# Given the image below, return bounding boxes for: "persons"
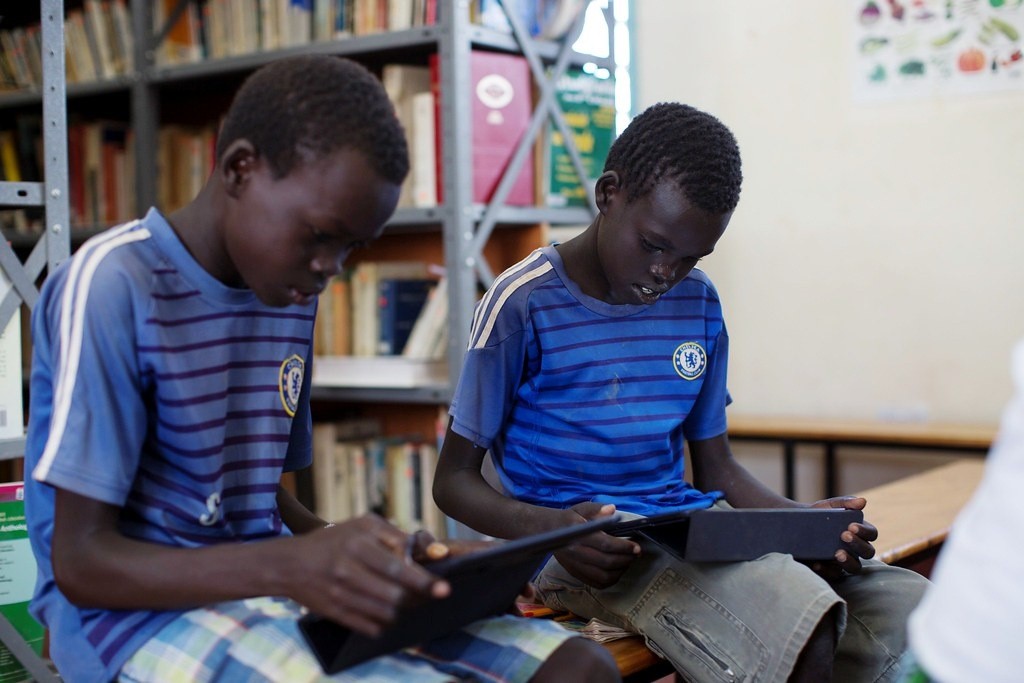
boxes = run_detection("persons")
[23,53,621,683]
[434,103,936,683]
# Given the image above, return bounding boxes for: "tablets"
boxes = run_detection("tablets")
[327,513,622,675]
[618,507,850,531]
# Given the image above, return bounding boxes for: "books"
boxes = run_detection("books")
[0,0,614,683]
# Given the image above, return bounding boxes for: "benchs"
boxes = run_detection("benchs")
[599,456,988,683]
[726,415,999,500]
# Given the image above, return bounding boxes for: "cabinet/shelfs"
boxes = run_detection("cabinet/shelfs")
[0,0,71,683]
[0,0,618,542]
